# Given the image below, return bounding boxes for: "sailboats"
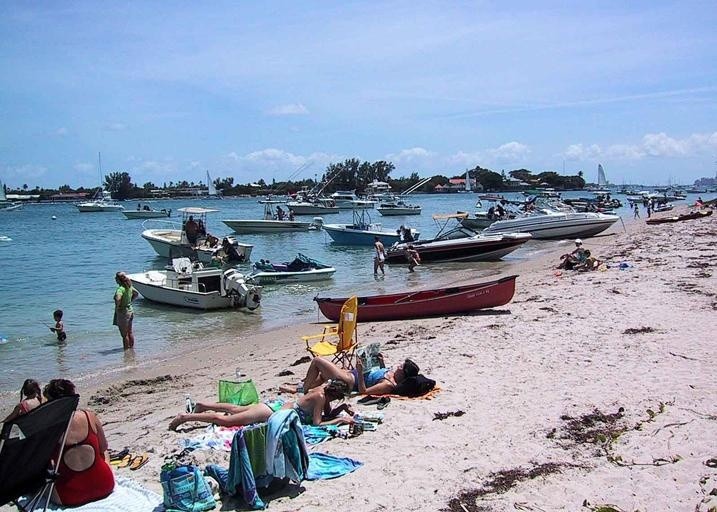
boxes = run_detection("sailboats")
[77,151,126,211]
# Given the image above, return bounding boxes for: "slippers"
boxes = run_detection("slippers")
[357,394,390,410]
[118,452,150,471]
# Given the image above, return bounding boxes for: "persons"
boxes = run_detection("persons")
[50,310,66,341]
[276,206,283,220]
[556,239,586,271]
[396,225,421,272]
[629,195,671,219]
[169,381,355,431]
[279,356,420,396]
[572,249,593,272]
[42,379,115,507]
[288,208,295,221]
[3,379,45,426]
[112,272,139,349]
[488,204,505,220]
[697,197,711,210]
[374,236,386,273]
[185,216,219,257]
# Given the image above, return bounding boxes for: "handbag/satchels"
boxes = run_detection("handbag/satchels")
[159,462,217,512]
[391,374,436,398]
[219,379,259,407]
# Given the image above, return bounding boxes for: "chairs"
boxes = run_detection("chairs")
[0,393,81,512]
[301,295,360,371]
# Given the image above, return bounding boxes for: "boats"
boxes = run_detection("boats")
[4,193,42,203]
[331,188,374,208]
[443,183,717,229]
[379,214,533,265]
[285,167,341,214]
[123,257,261,311]
[176,206,215,215]
[483,199,618,240]
[368,178,406,203]
[245,252,336,284]
[141,211,253,266]
[225,201,323,234]
[121,203,171,216]
[322,202,420,245]
[314,275,521,322]
